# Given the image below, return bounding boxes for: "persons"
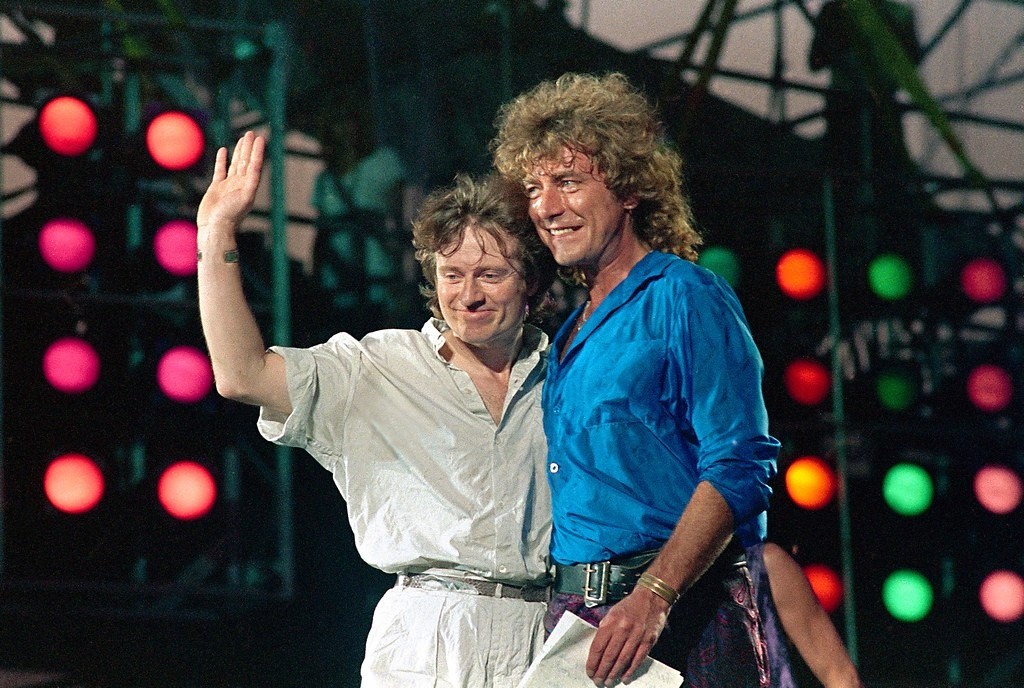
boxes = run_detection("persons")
[196,129,556,688]
[746,541,858,688]
[488,72,782,688]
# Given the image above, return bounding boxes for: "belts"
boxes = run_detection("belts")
[395,572,550,602]
[553,530,746,608]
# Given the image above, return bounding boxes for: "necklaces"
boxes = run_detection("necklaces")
[576,301,593,328]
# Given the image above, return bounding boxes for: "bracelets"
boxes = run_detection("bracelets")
[197,247,241,264]
[637,572,680,608]
